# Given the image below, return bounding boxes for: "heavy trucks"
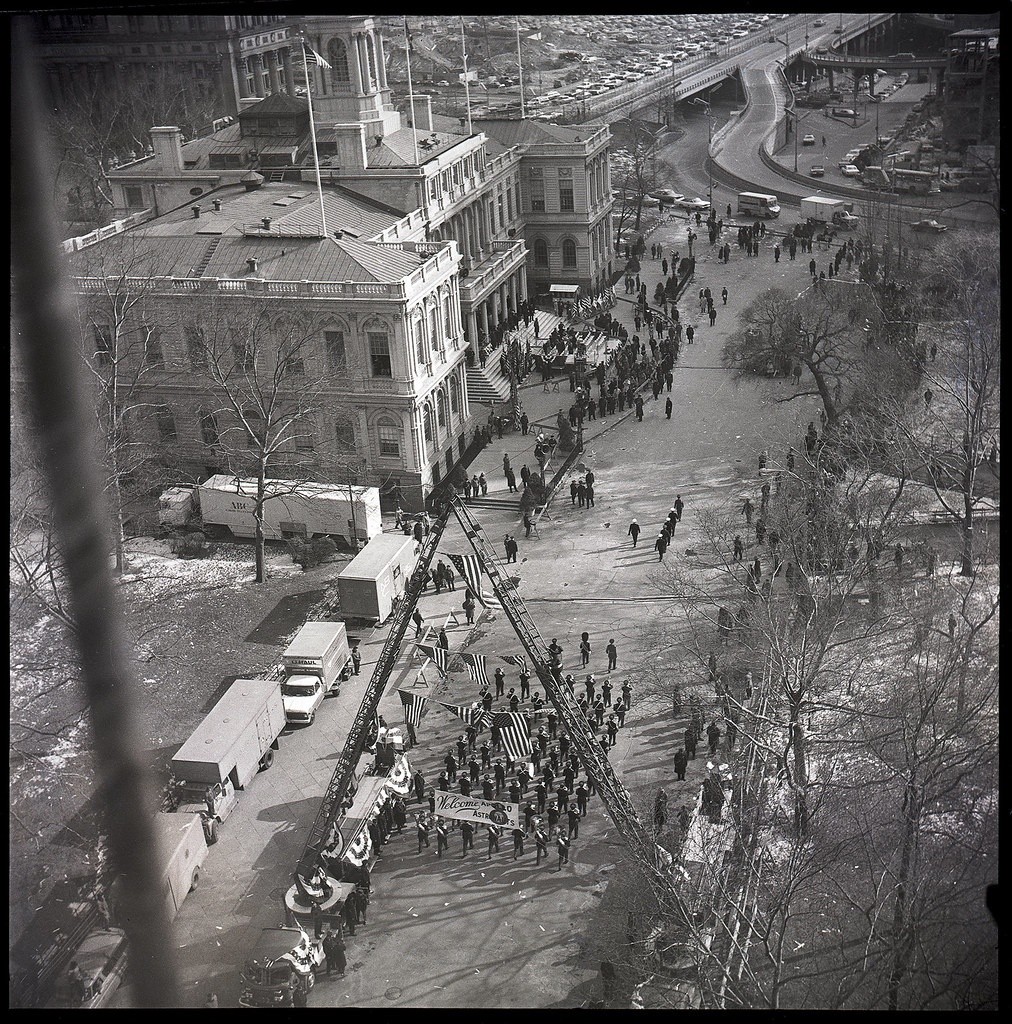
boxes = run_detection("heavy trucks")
[158,474,382,551]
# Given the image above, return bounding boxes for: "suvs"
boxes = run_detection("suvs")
[889,53,916,61]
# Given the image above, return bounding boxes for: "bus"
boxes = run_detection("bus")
[883,169,941,196]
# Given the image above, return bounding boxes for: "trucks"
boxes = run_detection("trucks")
[737,192,780,219]
[109,532,423,937]
[800,196,859,231]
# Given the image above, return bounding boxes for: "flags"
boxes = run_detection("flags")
[396,687,429,730]
[496,653,531,677]
[487,712,534,761]
[302,40,334,69]
[442,551,487,609]
[456,649,490,689]
[416,642,449,681]
[437,701,495,729]
[404,20,412,49]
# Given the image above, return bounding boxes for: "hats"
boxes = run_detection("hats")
[417,632,630,838]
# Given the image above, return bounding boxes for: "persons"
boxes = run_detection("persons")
[344,856,371,935]
[821,135,827,146]
[946,612,959,644]
[366,794,406,855]
[352,646,363,676]
[311,901,324,939]
[321,929,347,976]
[627,404,937,638]
[770,216,938,402]
[464,192,763,427]
[378,713,388,727]
[200,990,219,1007]
[406,555,725,875]
[205,785,218,819]
[389,410,595,636]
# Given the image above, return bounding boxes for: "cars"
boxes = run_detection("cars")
[835,109,858,117]
[523,51,673,118]
[648,190,683,203]
[909,220,947,234]
[625,195,659,207]
[839,143,881,182]
[674,197,710,211]
[879,92,933,147]
[802,134,814,145]
[810,165,824,176]
[828,69,908,99]
[468,14,845,59]
[388,75,513,97]
[42,927,128,1009]
